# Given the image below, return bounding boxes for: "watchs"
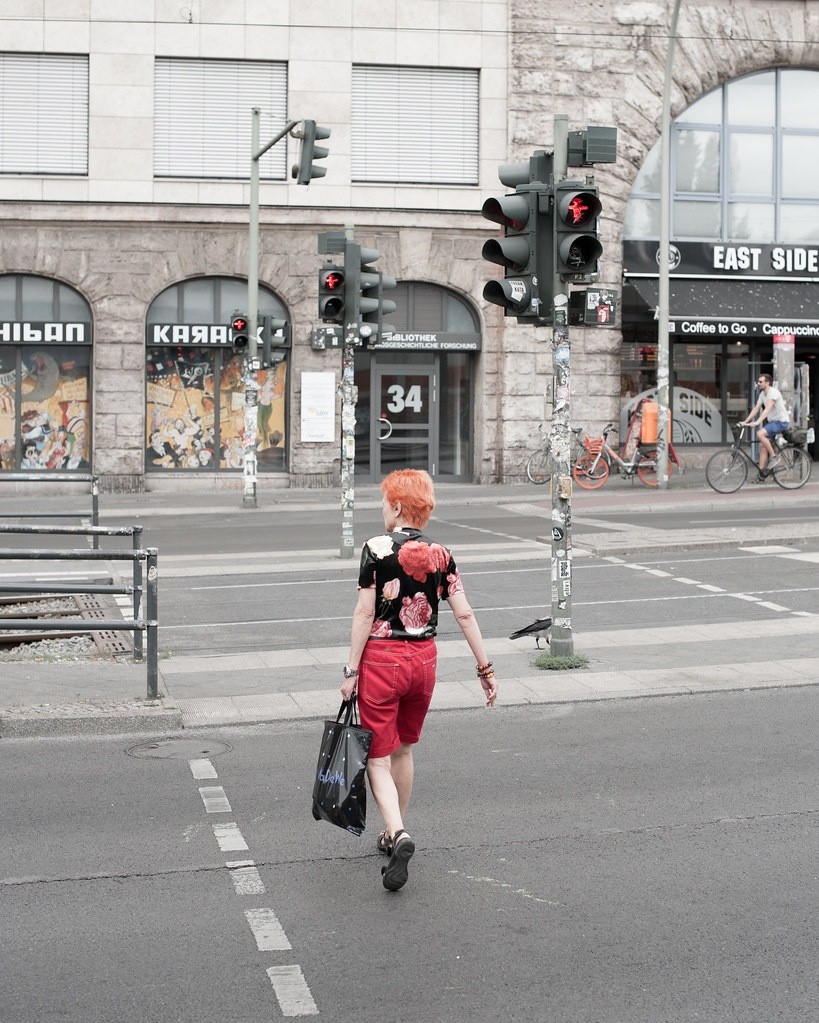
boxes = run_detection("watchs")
[343,666,359,678]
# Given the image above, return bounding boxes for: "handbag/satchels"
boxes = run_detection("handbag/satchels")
[311,692,373,836]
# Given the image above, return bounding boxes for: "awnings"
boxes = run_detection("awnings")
[628,277,819,337]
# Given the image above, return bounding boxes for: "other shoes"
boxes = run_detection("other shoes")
[767,455,781,470]
[752,477,764,484]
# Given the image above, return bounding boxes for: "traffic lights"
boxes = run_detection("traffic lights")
[363,269,395,345]
[290,119,328,186]
[551,175,603,276]
[260,315,288,369]
[481,150,540,319]
[230,310,247,354]
[345,242,379,329]
[318,264,344,320]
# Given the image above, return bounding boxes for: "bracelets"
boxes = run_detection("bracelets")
[475,662,494,677]
[755,421,760,426]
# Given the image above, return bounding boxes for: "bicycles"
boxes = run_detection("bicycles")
[573,424,679,490]
[705,421,813,495]
[526,425,611,483]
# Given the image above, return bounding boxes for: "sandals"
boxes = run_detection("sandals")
[381,830,415,891]
[376,831,396,858]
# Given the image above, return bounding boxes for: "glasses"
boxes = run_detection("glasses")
[757,380,764,384]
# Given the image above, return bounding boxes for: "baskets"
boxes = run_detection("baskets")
[585,434,606,454]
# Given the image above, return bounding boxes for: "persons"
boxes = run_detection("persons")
[741,374,790,484]
[340,469,499,891]
